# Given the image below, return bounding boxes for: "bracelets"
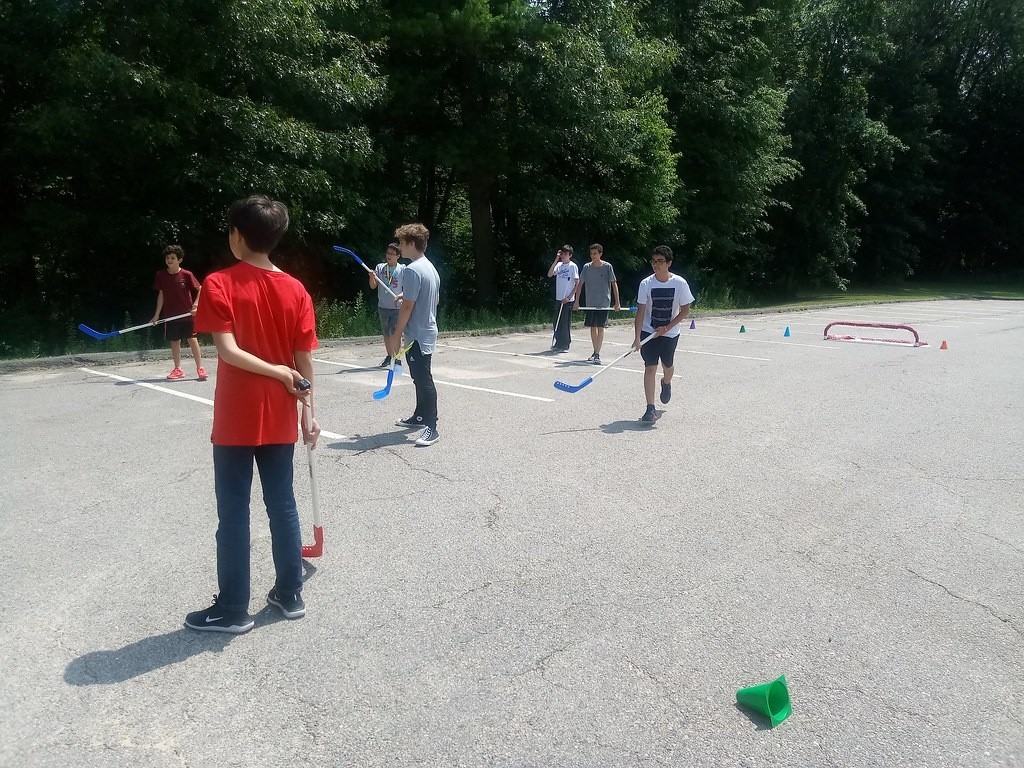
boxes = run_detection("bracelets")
[665,326,668,332]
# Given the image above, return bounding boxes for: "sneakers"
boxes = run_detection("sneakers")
[593,354,601,365]
[660,377,671,404]
[642,404,658,421]
[268,586,306,618]
[558,345,569,352]
[184,594,255,633]
[167,368,185,379]
[415,428,440,446]
[196,367,208,379]
[396,413,425,428]
[588,353,601,361]
[551,345,560,351]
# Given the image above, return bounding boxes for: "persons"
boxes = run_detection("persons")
[631,246,695,422]
[388,224,441,446]
[369,244,406,366]
[150,245,208,378]
[573,244,620,364]
[185,195,321,632]
[548,246,580,352]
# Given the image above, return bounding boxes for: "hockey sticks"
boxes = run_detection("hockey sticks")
[332,245,401,304]
[551,276,570,352]
[577,305,638,313]
[554,330,657,394]
[298,378,325,558]
[78,312,191,341]
[372,355,395,400]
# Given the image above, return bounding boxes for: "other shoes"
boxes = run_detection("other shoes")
[382,356,391,365]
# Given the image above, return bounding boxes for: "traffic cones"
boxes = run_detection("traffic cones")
[940,340,948,349]
[689,320,695,329]
[736,675,792,729]
[784,327,791,336]
[739,325,746,332]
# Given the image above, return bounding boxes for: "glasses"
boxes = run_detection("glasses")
[386,252,398,256]
[650,259,666,265]
